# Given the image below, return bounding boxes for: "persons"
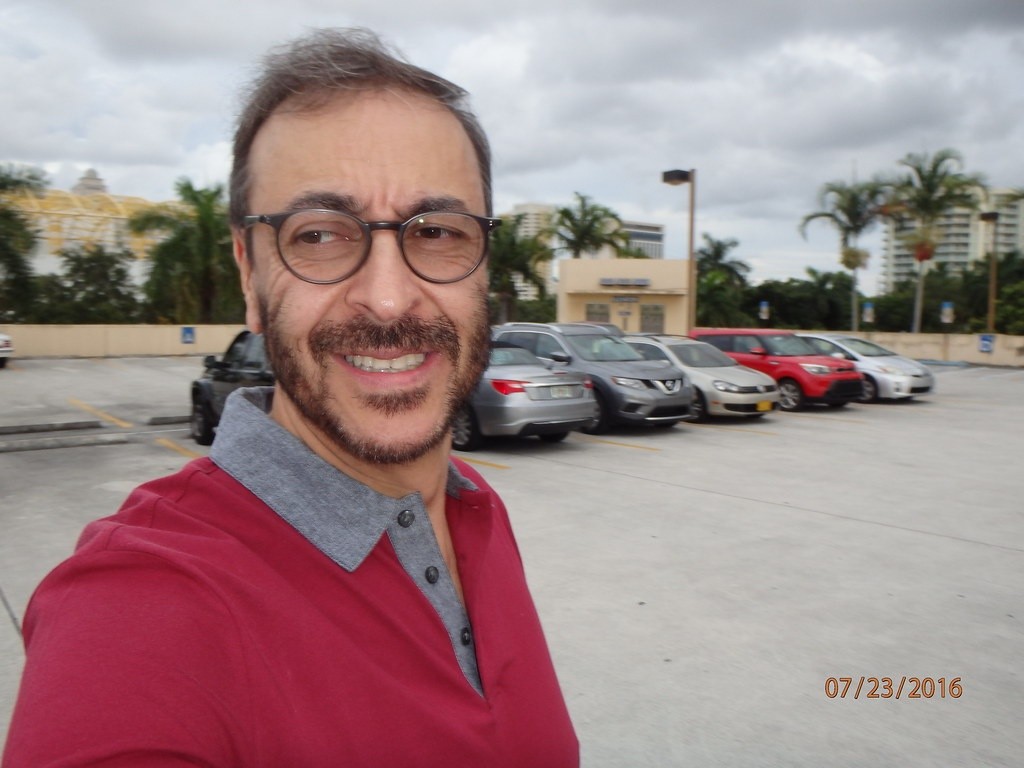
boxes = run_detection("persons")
[1,32,580,767]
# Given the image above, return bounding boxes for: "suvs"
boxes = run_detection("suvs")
[489,320,698,434]
[684,326,865,410]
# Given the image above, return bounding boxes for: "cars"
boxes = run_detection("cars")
[186,327,276,445]
[450,340,596,452]
[795,330,934,406]
[594,332,783,423]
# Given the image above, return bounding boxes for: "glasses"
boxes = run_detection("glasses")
[242,207,503,284]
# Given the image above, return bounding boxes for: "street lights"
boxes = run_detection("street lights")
[661,169,697,339]
